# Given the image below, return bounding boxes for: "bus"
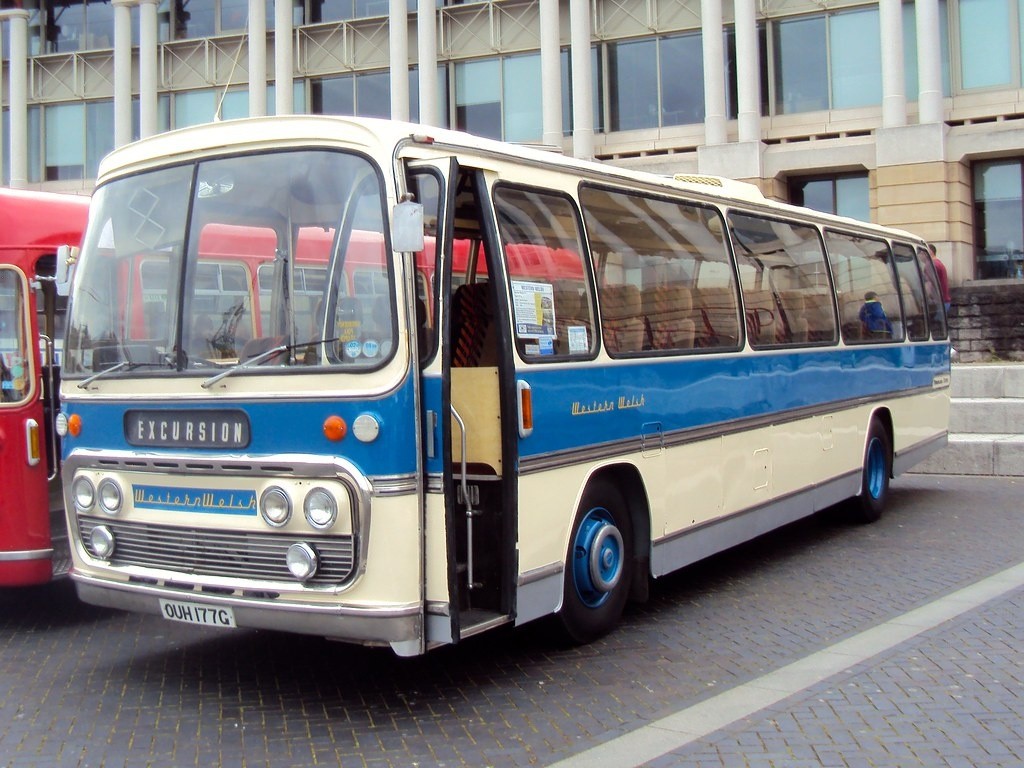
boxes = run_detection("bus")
[0,187,612,616]
[50,111,956,665]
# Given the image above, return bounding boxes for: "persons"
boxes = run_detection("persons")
[858,291,895,339]
[919,244,951,321]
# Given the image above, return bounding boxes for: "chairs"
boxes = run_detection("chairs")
[449,283,852,365]
[238,297,432,366]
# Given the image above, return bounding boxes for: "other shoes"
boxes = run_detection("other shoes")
[950,347,957,358]
